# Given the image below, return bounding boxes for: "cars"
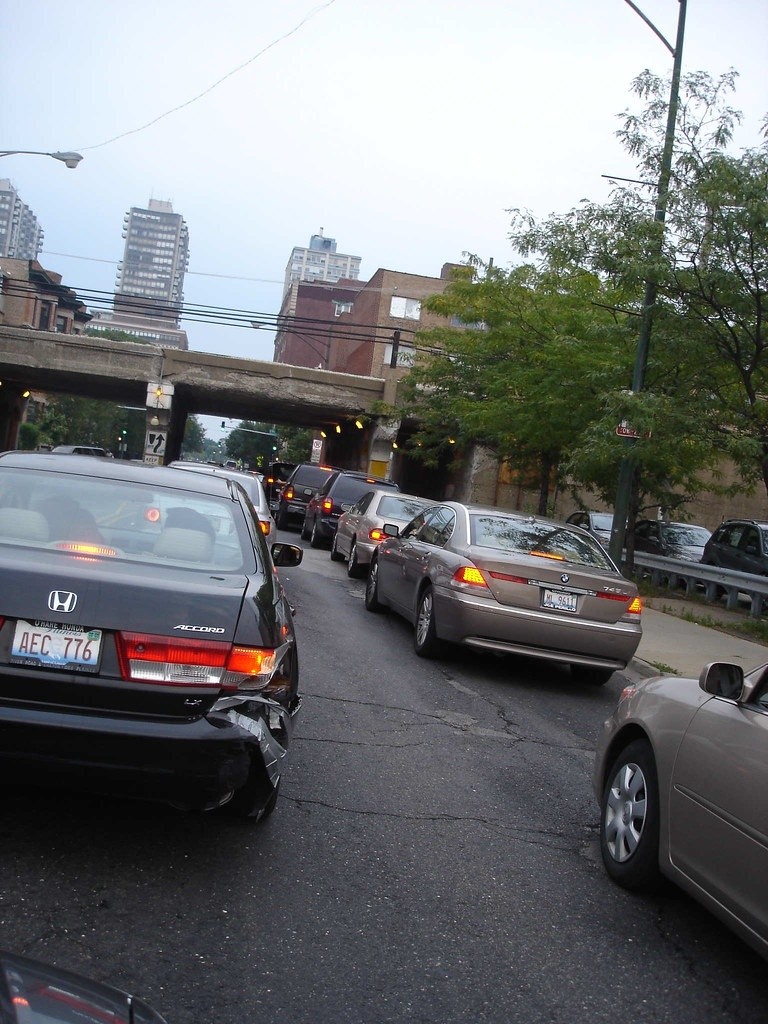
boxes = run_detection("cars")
[140,460,277,574]
[366,501,643,684]
[331,489,443,578]
[593,663,768,963]
[623,518,713,587]
[1,448,304,823]
[566,510,614,549]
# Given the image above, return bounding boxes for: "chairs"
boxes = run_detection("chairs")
[746,535,767,556]
[152,528,215,563]
[0,507,51,543]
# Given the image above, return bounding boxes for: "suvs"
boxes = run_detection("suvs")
[51,445,108,458]
[701,518,768,611]
[300,469,400,548]
[207,460,343,529]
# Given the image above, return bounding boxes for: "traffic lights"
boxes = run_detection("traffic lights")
[221,421,225,432]
[219,450,222,454]
[217,441,222,448]
[271,440,278,454]
[122,424,128,436]
[213,450,216,455]
[117,435,122,442]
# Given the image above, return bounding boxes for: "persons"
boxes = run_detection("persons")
[163,506,216,545]
